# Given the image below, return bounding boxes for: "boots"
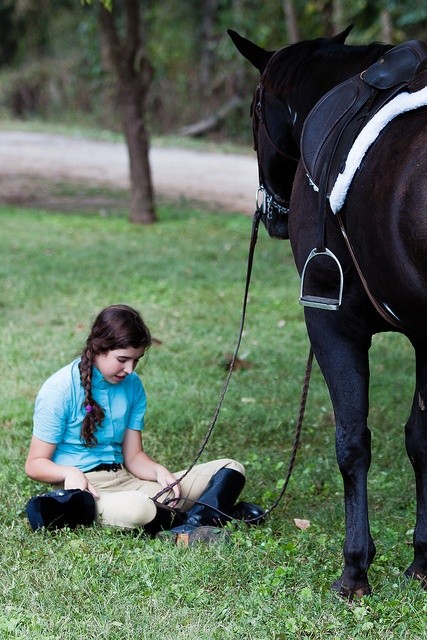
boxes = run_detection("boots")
[154,467,246,550]
[133,497,266,534]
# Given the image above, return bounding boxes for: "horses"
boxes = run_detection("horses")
[226,21,427,601]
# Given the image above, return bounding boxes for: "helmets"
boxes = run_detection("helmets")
[25,489,95,532]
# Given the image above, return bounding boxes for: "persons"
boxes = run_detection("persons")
[24,303,264,543]
